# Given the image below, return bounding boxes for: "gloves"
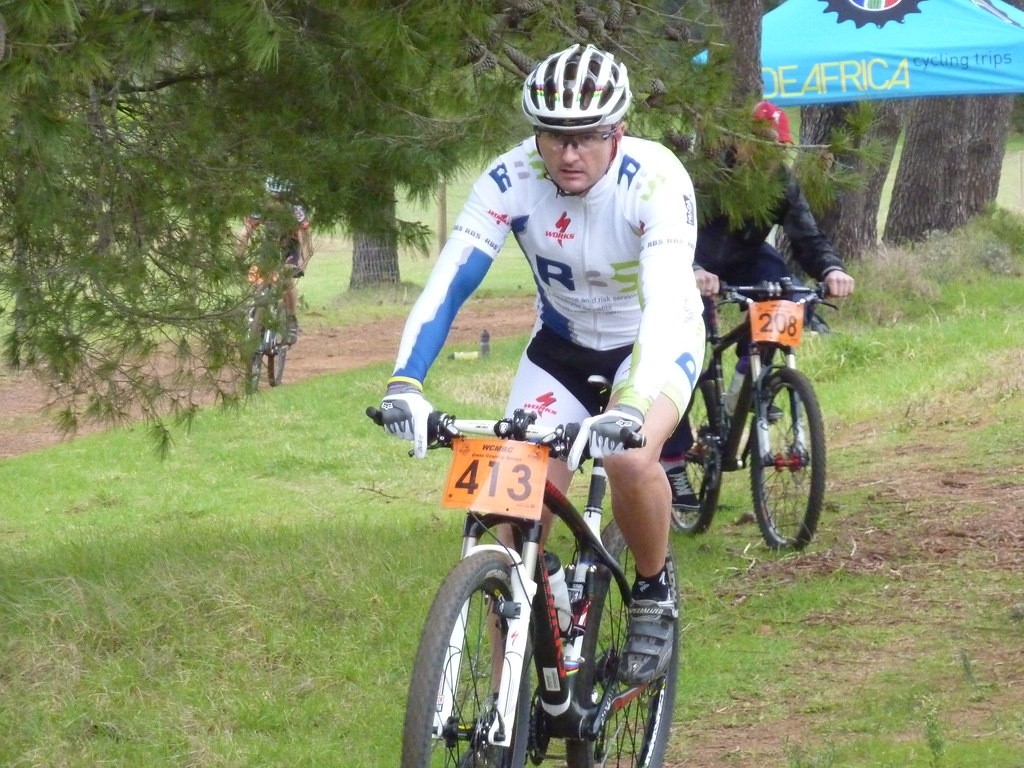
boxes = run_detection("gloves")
[567,404,644,471]
[380,382,433,459]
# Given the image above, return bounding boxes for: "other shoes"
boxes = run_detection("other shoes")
[286,324,298,344]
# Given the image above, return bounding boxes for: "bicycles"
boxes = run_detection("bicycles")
[244,258,304,396]
[365,374,680,768]
[656,277,841,553]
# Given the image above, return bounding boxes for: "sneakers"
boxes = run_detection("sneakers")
[666,466,700,509]
[458,748,474,768]
[621,589,678,685]
[750,400,784,419]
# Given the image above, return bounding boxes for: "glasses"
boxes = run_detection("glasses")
[534,126,617,154]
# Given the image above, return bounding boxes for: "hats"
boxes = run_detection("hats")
[751,102,793,144]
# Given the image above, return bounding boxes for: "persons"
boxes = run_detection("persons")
[237,172,309,348]
[660,100,855,512]
[382,42,708,686]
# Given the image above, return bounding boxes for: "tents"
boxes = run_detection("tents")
[646,0,1024,108]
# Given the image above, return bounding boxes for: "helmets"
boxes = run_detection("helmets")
[522,44,632,130]
[265,173,295,194]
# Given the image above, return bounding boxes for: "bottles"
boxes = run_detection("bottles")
[543,549,573,635]
[447,351,479,360]
[725,353,749,416]
[560,595,588,665]
[480,329,490,358]
